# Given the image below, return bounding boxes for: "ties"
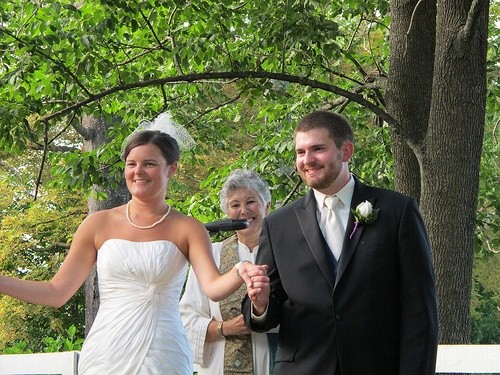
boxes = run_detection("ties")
[324,196,343,261]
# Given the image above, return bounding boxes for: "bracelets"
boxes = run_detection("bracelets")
[217,322,225,339]
[236,260,252,277]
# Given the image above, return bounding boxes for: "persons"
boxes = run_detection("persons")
[0,111,264,375]
[178,169,271,375]
[248,110,438,375]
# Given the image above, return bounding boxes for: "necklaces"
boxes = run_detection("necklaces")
[124,200,171,229]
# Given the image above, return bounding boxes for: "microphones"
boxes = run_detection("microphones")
[204,218,249,233]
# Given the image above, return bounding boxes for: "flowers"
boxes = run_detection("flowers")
[350,200,381,239]
